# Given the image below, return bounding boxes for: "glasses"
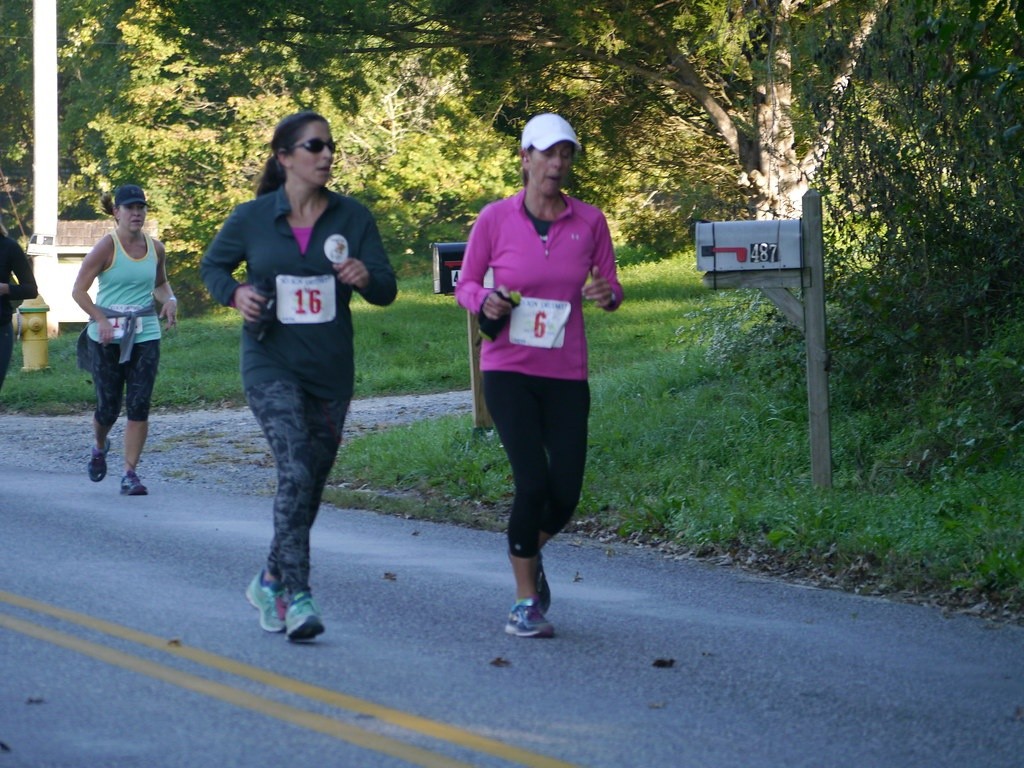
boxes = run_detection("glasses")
[287,139,335,153]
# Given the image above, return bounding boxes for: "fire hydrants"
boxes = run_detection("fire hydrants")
[10,292,53,373]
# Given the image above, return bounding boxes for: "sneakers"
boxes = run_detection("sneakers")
[245,568,289,633]
[508,546,551,614]
[120,470,147,495]
[505,593,554,638]
[285,591,325,641]
[87,437,110,482]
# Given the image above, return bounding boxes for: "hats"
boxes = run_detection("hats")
[522,114,581,152]
[115,185,150,207]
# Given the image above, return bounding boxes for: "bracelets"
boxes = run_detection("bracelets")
[603,290,617,309]
[169,297,176,301]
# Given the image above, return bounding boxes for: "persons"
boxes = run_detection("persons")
[72,184,178,495]
[455,113,624,638]
[0,224,38,392]
[198,112,398,644]
